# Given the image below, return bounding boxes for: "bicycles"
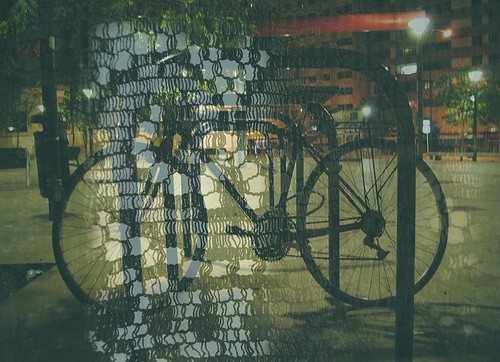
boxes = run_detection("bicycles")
[48,53,448,315]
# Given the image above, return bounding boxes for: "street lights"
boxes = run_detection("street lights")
[408,18,427,164]
[466,66,483,162]
[429,28,453,155]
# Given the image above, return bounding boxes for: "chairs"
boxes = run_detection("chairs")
[0,147,32,188]
